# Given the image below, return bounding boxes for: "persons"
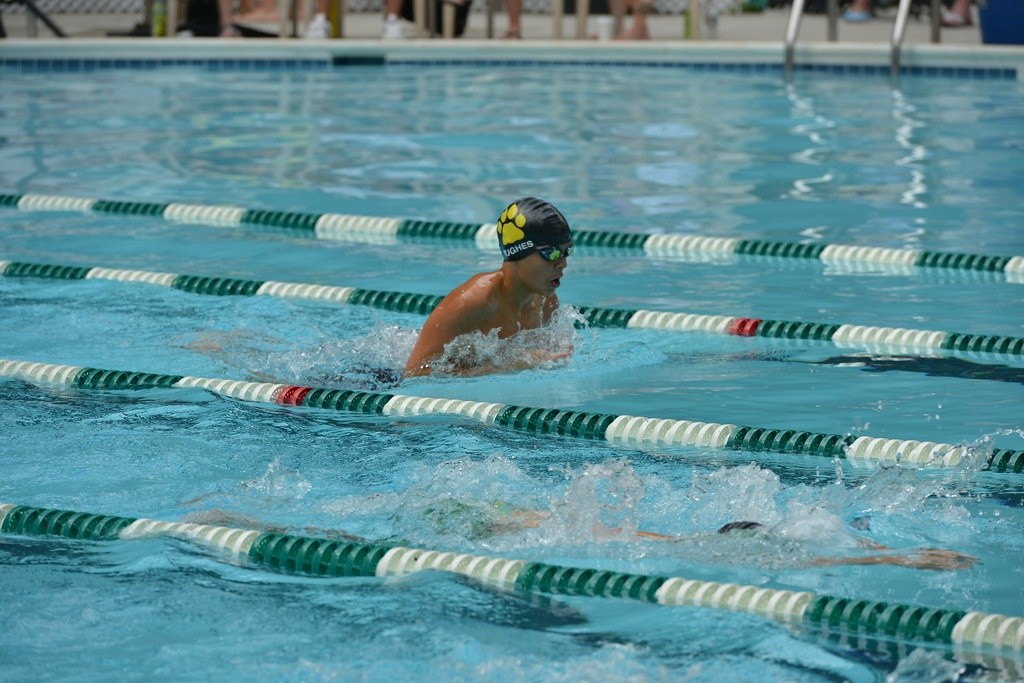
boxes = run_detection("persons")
[169,491,981,571]
[176,196,574,382]
[0,0,972,41]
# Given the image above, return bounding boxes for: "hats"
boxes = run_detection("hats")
[496,197,572,261]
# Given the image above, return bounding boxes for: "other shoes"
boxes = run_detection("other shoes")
[382,13,408,39]
[307,13,334,37]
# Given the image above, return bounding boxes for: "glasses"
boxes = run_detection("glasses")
[535,243,575,261]
